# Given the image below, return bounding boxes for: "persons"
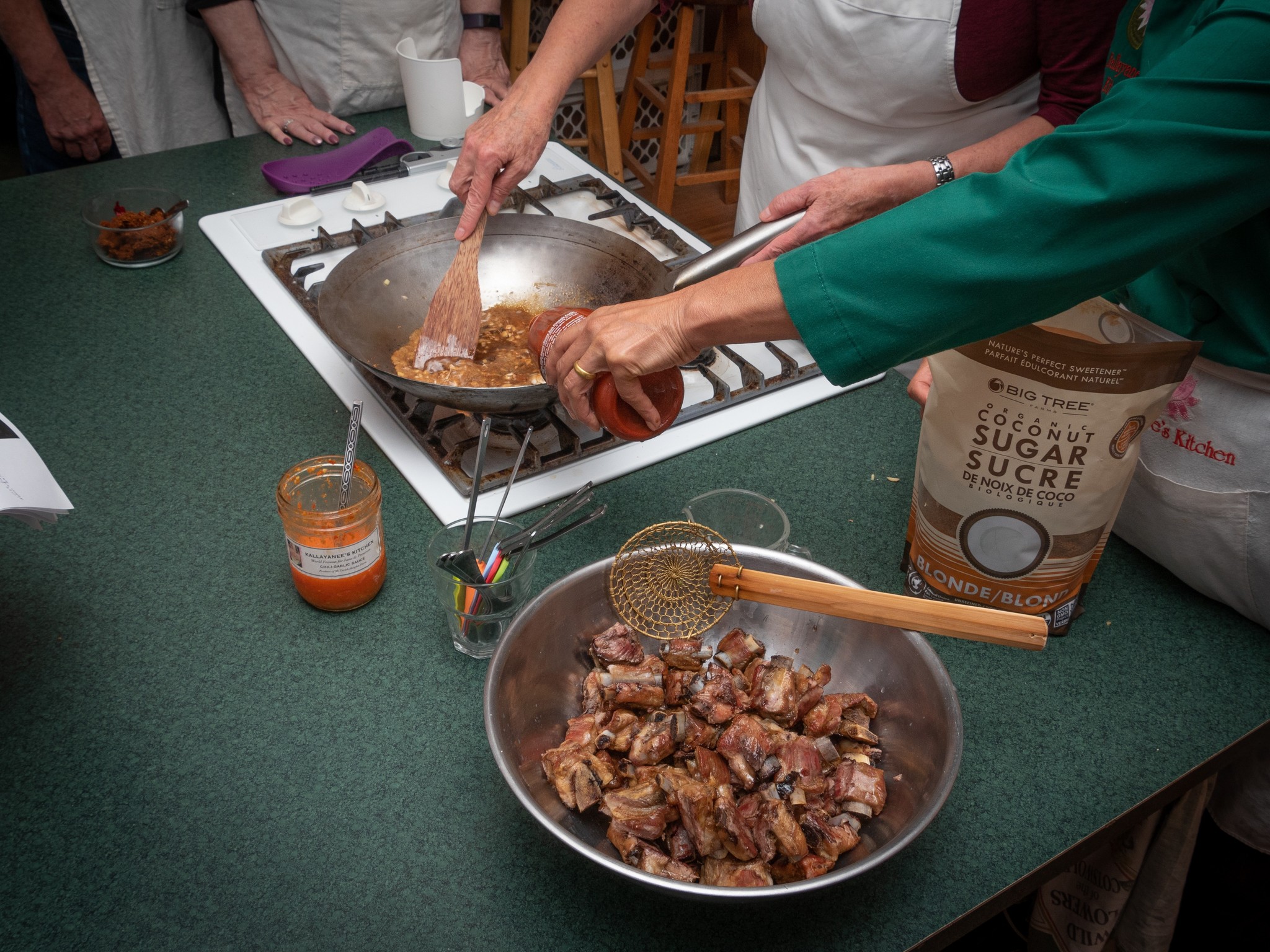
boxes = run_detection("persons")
[544,1,1270,952]
[0,0,514,178]
[450,0,1124,270]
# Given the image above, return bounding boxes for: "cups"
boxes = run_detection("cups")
[681,488,813,562]
[427,516,538,659]
[276,455,387,611]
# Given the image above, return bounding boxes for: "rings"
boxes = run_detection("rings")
[574,359,597,379]
[284,118,294,133]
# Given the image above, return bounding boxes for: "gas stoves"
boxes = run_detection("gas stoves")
[198,141,887,529]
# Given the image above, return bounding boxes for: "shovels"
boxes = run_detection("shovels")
[413,208,487,370]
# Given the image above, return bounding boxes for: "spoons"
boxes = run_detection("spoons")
[149,199,190,223]
[435,549,519,647]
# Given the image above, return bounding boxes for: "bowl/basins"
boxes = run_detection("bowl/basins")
[483,542,963,897]
[79,187,186,268]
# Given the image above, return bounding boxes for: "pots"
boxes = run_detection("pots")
[317,208,808,413]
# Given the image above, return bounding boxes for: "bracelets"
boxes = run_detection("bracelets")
[462,11,501,29]
[927,155,955,186]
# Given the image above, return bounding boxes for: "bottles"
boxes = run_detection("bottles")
[527,305,685,442]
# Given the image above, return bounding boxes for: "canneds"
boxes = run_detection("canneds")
[276,455,387,612]
